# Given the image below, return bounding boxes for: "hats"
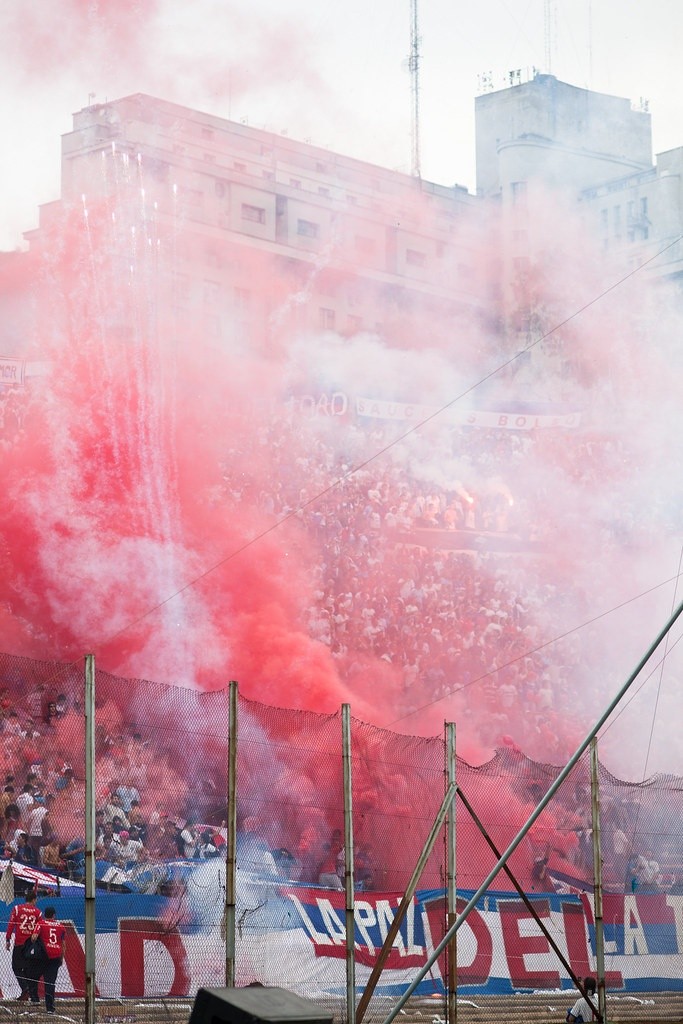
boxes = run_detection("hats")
[129,825,142,832]
[534,856,543,865]
[119,831,130,839]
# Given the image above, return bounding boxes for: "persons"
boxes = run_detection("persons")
[566,977,598,1024]
[1,429,682,891]
[5,891,68,1013]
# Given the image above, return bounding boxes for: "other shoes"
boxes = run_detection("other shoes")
[47,1007,54,1014]
[30,999,41,1005]
[17,992,28,1001]
